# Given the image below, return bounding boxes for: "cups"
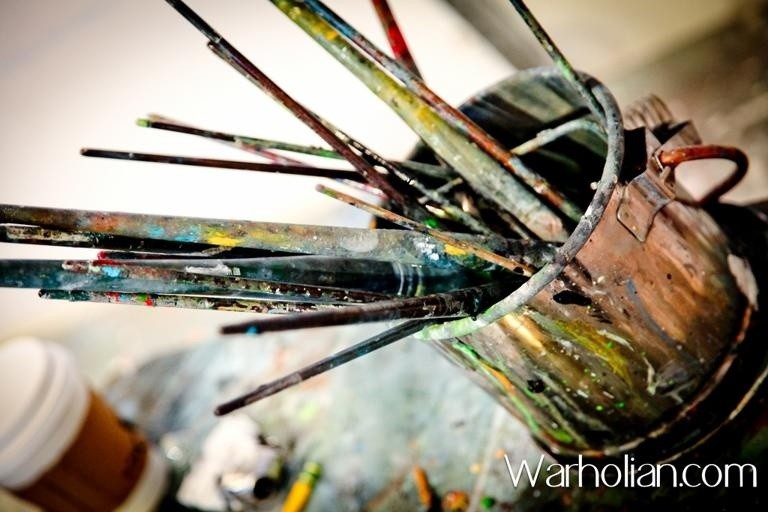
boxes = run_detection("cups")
[364,64,768,482]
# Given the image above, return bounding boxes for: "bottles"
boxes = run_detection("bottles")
[0,338,170,512]
